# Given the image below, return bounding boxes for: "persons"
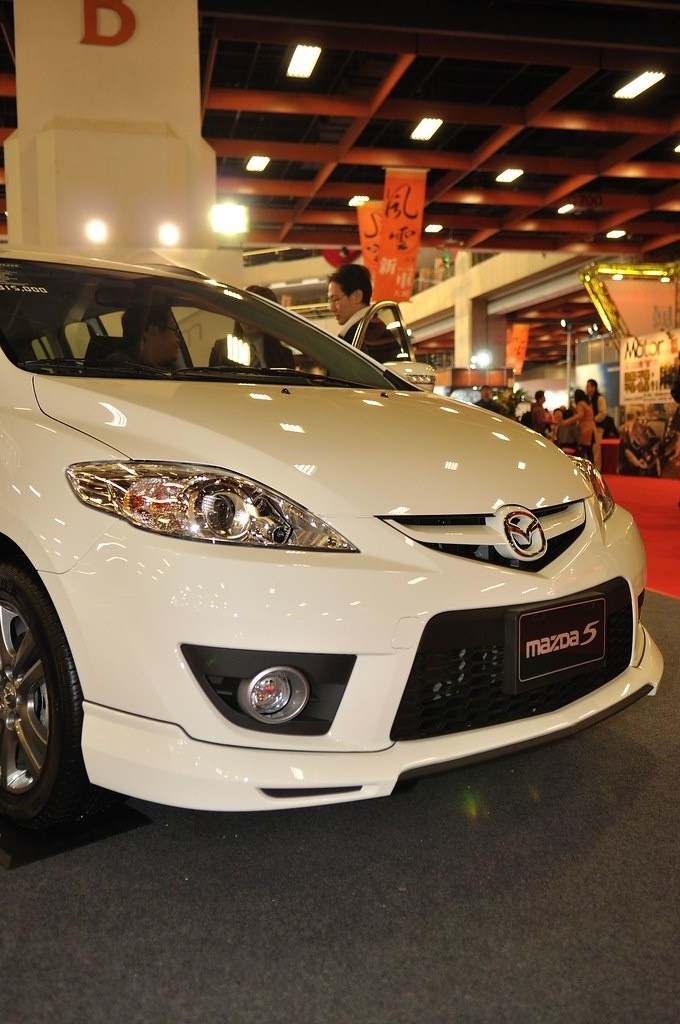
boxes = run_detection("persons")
[560,389,597,464]
[327,264,404,362]
[208,284,295,369]
[671,370,680,434]
[531,391,550,435]
[619,416,656,476]
[109,301,180,369]
[473,385,502,413]
[544,406,574,432]
[586,379,618,439]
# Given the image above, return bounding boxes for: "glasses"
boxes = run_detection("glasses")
[328,293,346,303]
[167,325,180,338]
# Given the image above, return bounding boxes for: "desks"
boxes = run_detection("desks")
[601,437,623,475]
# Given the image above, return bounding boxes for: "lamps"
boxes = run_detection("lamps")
[561,318,569,329]
[345,194,372,211]
[493,164,527,185]
[243,151,273,176]
[587,322,600,337]
[279,36,325,86]
[608,66,670,104]
[406,113,445,142]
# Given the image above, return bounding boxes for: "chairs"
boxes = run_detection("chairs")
[555,426,590,463]
[82,338,137,377]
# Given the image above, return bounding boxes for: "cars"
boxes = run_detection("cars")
[0,249,664,827]
